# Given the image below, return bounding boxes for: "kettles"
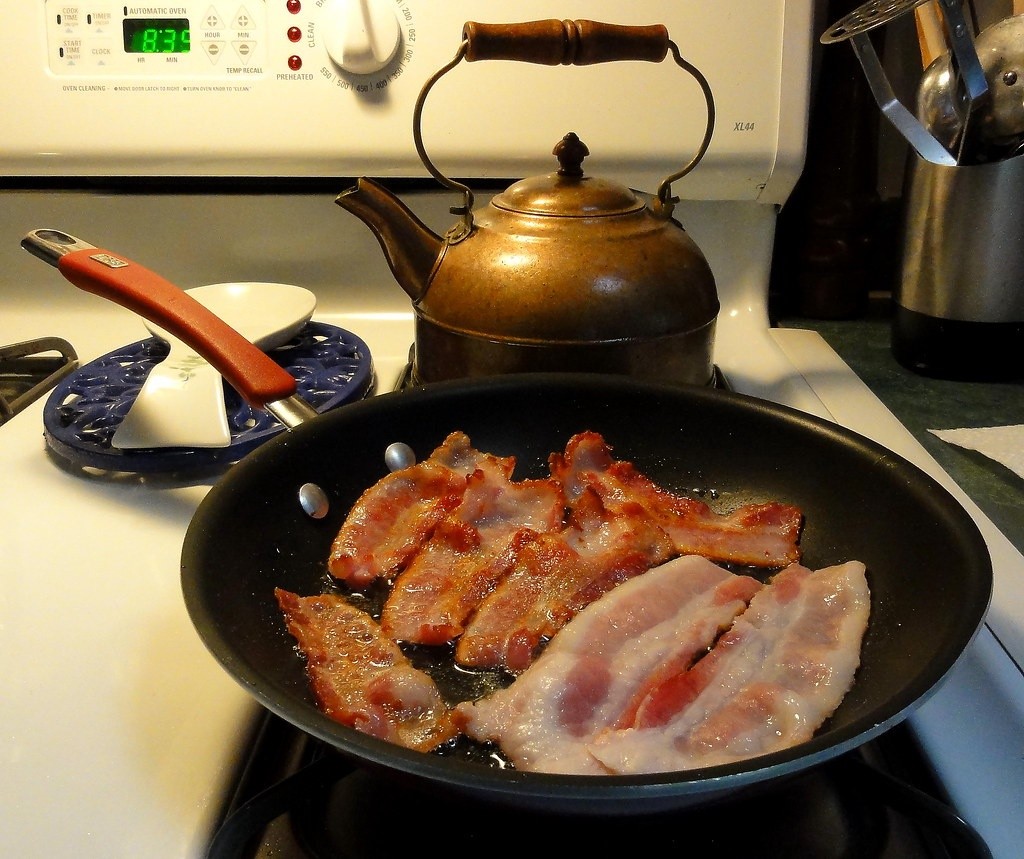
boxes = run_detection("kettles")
[333,21,721,387]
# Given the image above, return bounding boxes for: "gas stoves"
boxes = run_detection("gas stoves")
[0,0,1024,859]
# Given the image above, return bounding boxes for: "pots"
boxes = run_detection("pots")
[19,229,991,816]
